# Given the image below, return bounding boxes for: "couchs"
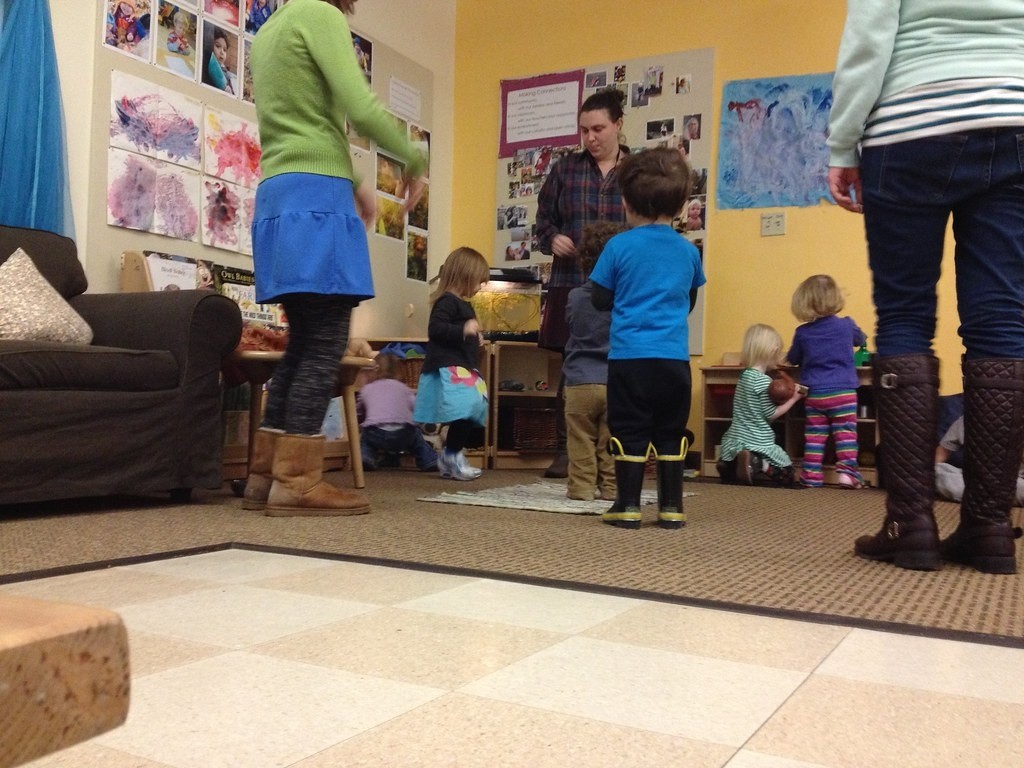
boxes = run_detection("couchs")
[0,224,243,507]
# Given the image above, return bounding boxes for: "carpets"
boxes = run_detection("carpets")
[0,457,1024,648]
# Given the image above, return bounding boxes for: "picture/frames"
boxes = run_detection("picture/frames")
[102,0,433,284]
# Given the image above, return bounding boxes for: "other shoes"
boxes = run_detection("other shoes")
[715,449,763,486]
[835,473,863,489]
[437,444,481,481]
[544,452,568,478]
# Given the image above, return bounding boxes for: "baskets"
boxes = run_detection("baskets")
[397,358,425,388]
[511,403,561,453]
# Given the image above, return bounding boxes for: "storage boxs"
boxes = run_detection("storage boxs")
[399,358,424,388]
[511,405,558,449]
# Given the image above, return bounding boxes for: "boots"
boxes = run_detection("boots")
[854,353,941,570]
[940,353,1024,574]
[601,436,686,529]
[243,426,371,517]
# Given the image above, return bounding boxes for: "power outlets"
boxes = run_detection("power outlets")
[761,211,787,236]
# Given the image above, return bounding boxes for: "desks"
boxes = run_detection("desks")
[238,350,376,488]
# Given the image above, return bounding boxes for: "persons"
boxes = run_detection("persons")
[826,0,1024,575]
[785,274,869,490]
[715,323,808,488]
[241,1,430,518]
[498,64,705,263]
[352,38,433,280]
[535,86,707,527]
[355,352,439,473]
[106,0,278,100]
[934,414,1024,509]
[412,247,492,482]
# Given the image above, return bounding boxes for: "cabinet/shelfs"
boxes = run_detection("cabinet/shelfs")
[699,364,881,489]
[492,334,571,474]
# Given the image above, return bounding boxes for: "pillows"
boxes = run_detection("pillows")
[0,246,95,345]
